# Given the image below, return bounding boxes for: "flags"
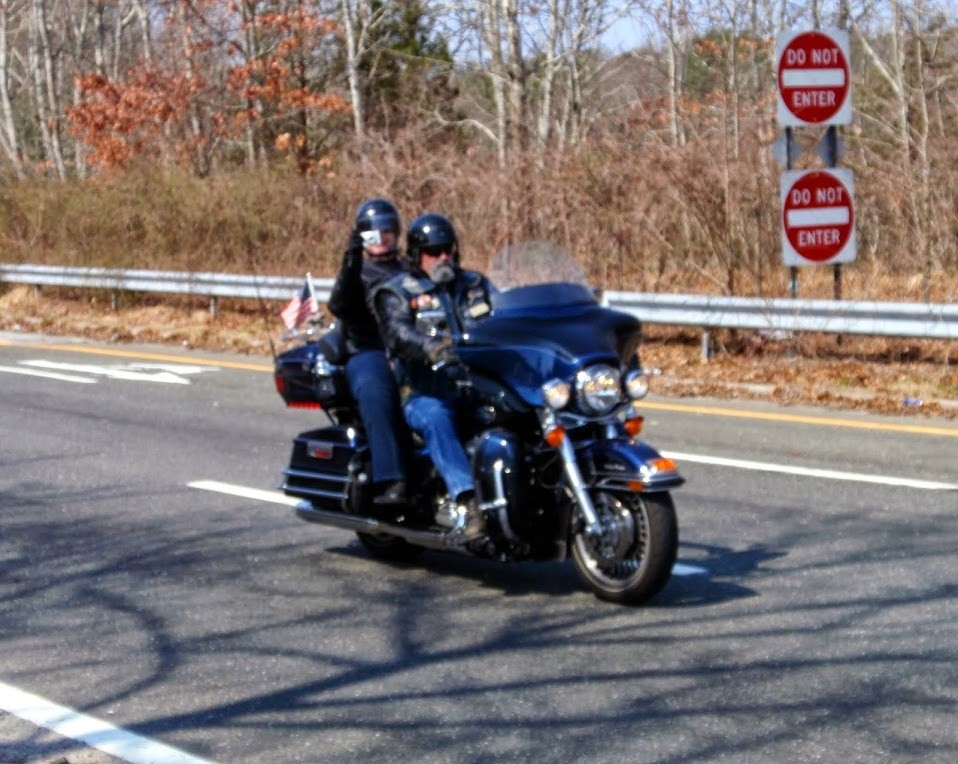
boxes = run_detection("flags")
[279,278,323,335]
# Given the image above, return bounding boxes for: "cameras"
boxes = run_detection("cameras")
[359,229,382,247]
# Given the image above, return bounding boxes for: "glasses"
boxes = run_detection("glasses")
[423,244,456,257]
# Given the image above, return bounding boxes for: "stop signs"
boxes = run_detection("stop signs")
[773,27,853,128]
[778,168,858,264]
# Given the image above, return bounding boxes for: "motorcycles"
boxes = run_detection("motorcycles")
[274,243,686,607]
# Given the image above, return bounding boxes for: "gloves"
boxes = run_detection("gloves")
[422,338,458,367]
[347,230,364,271]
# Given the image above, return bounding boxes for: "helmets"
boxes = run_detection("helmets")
[355,200,400,231]
[406,213,460,258]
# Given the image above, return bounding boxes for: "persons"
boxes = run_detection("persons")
[327,198,426,500]
[369,214,505,544]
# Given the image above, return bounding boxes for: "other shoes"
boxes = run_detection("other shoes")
[454,496,487,545]
[383,480,408,500]
